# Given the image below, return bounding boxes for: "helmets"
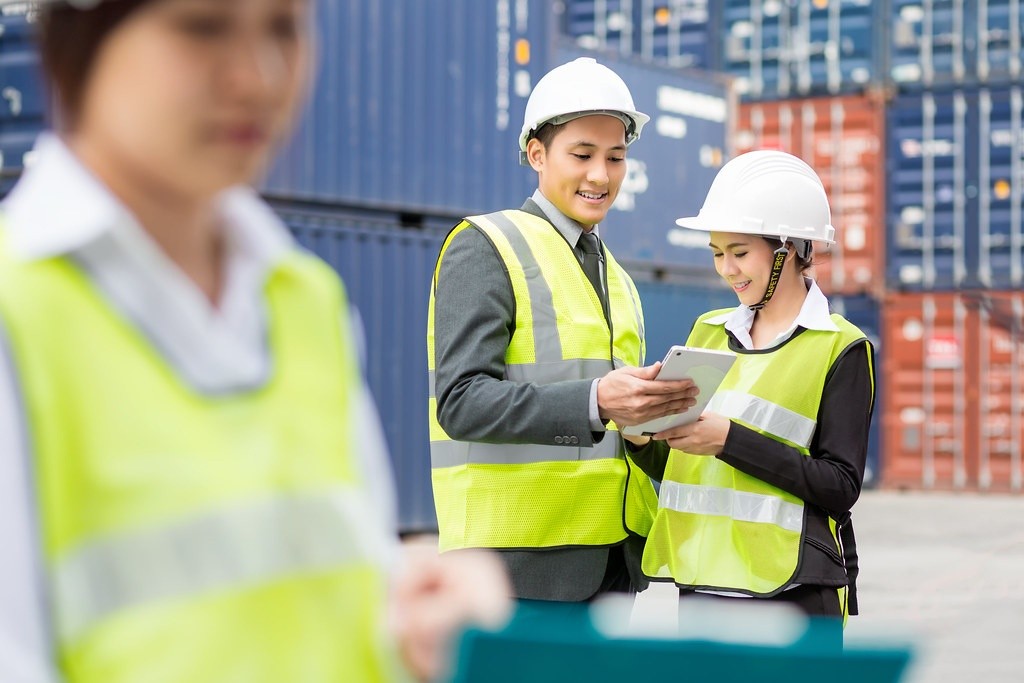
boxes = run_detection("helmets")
[519,57,650,155]
[676,148,836,246]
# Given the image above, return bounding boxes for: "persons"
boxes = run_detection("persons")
[426,57,701,629]
[614,148,876,656]
[2,2,516,681]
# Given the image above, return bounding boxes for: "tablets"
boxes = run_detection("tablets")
[621,345,736,437]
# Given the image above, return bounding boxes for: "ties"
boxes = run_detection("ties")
[577,233,607,322]
